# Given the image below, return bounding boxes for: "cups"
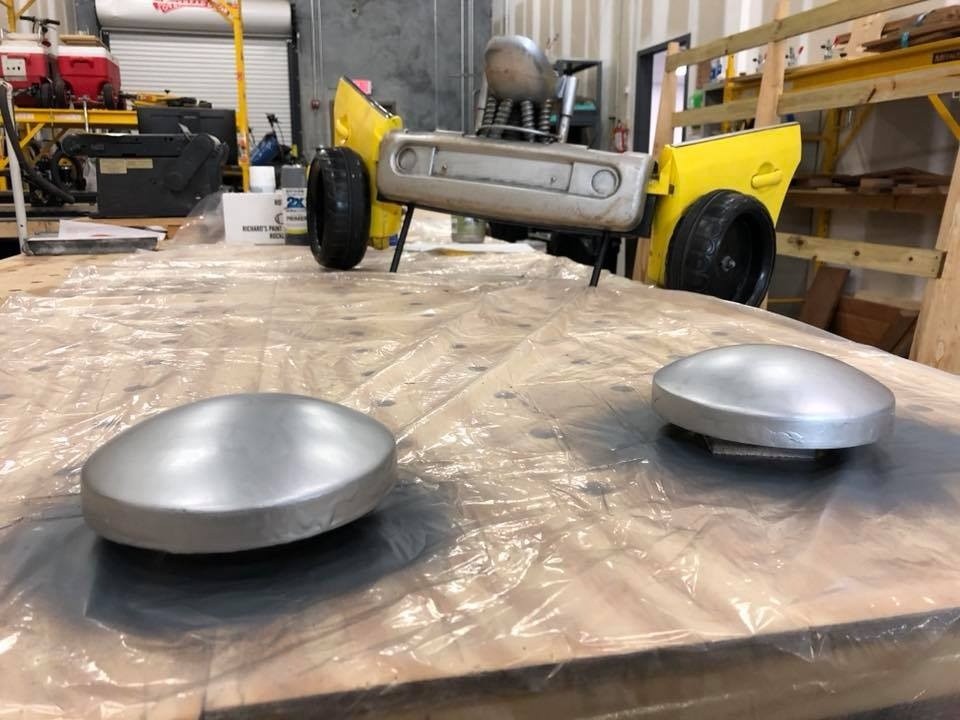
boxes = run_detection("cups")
[452,213,486,242]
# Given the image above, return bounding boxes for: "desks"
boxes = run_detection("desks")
[0,239,960,720]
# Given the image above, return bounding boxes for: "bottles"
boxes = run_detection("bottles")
[281,155,310,245]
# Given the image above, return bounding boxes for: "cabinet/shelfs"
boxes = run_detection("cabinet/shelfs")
[634,0,960,378]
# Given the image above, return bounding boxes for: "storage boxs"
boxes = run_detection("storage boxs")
[0,45,49,89]
[57,45,121,106]
